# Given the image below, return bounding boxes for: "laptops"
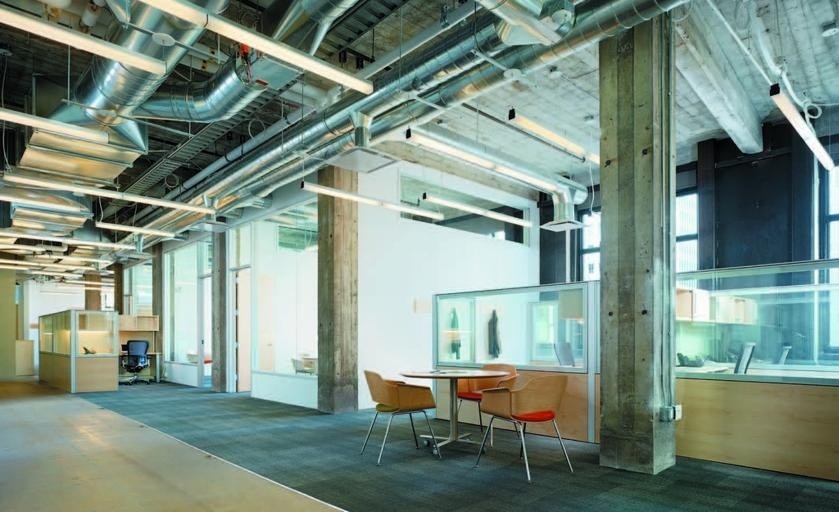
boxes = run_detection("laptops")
[121,343,128,354]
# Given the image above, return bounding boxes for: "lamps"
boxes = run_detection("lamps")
[768,76,837,178]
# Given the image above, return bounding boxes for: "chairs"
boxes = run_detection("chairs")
[121,339,151,385]
[358,363,577,487]
[732,341,757,374]
[774,346,791,365]
[553,343,576,365]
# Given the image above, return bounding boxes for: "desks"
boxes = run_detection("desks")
[119,352,164,385]
